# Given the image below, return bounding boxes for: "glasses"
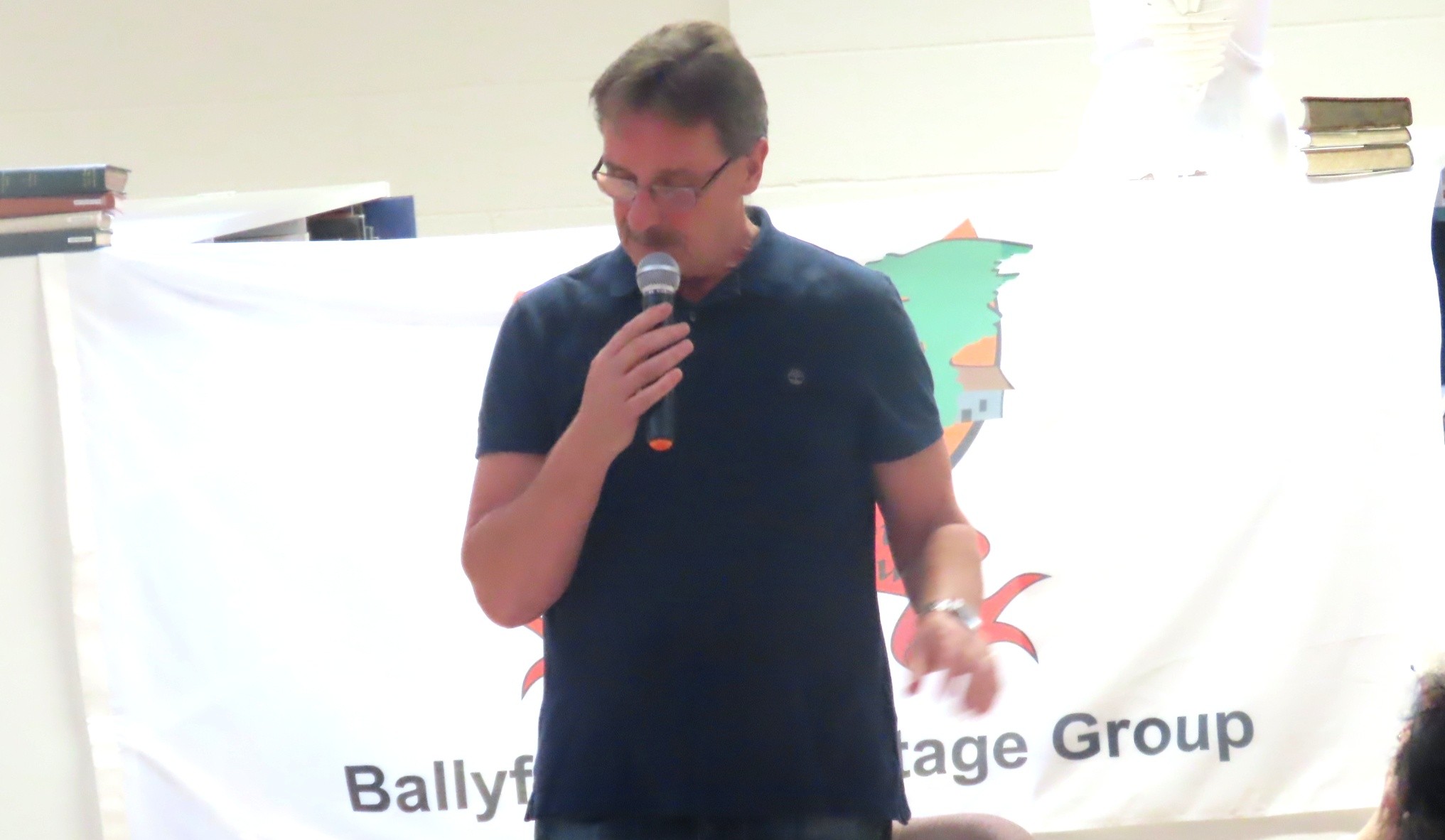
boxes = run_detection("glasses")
[592,147,735,208]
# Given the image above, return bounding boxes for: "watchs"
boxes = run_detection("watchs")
[913,599,986,630]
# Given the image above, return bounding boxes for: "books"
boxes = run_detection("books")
[0,164,133,258]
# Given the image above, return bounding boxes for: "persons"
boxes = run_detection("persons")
[461,22,999,840]
[1359,669,1445,840]
[893,813,1031,840]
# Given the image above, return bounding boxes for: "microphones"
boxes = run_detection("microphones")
[635,253,681,452]
